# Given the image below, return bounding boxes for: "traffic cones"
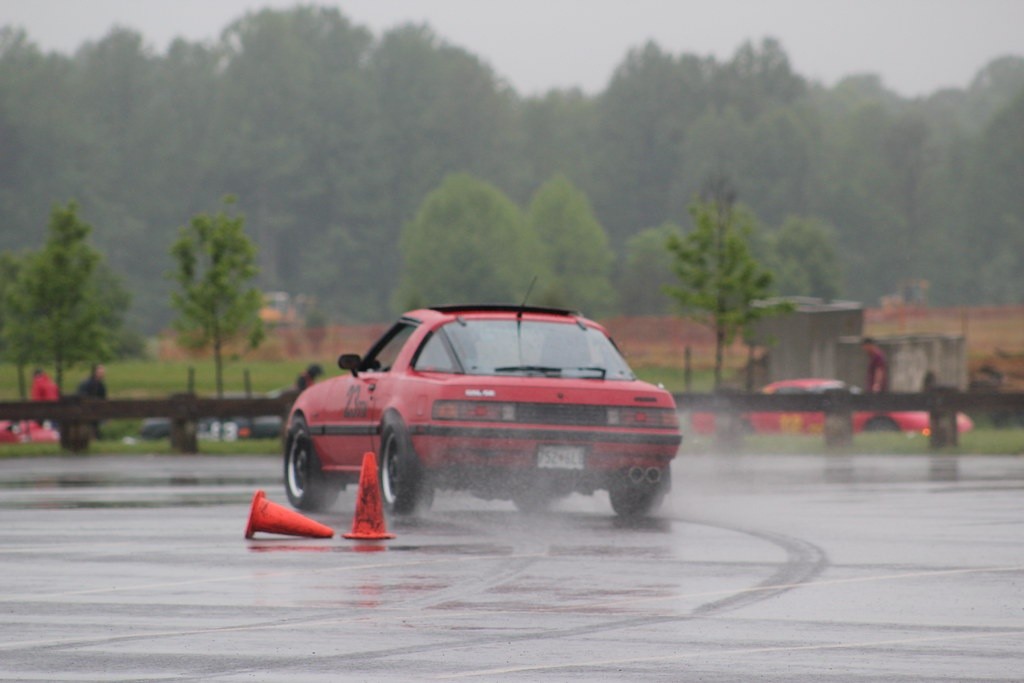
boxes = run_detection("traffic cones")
[341,450,396,541]
[243,490,336,539]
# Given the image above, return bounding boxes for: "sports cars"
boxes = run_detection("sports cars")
[283,302,684,520]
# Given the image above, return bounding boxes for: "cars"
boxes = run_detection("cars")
[138,387,295,442]
[690,377,974,440]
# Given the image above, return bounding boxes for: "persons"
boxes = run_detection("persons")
[32,368,59,400]
[77,364,105,398]
[861,339,886,393]
[292,365,323,392]
